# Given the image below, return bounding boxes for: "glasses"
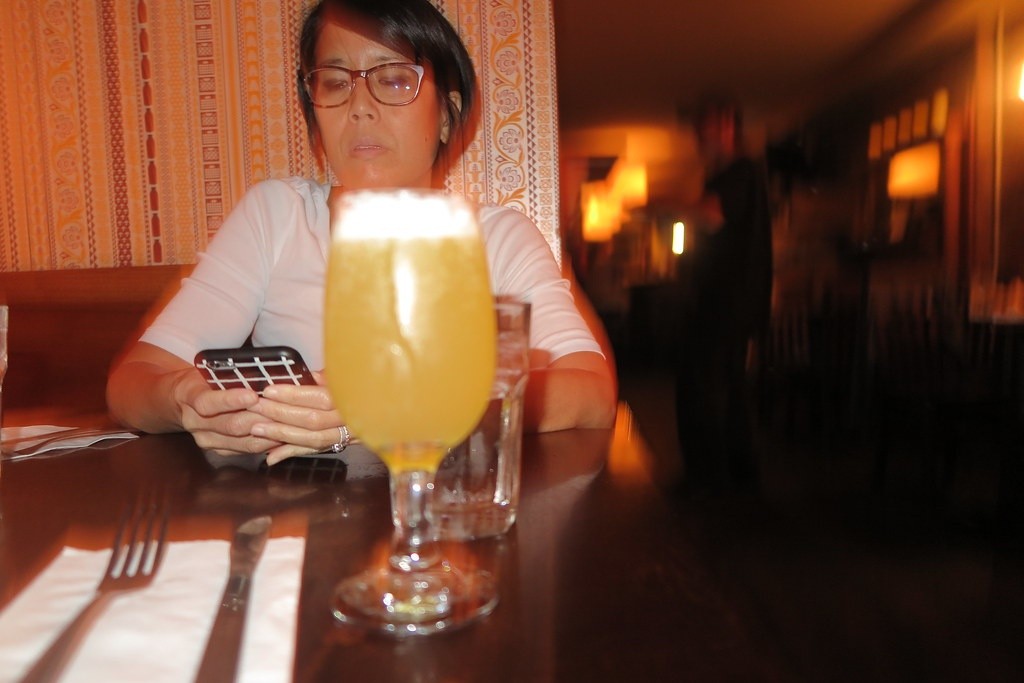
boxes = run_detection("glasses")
[302,63,447,107]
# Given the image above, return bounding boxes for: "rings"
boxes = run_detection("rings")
[332,427,350,453]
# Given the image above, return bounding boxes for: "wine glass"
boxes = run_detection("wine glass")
[322,190,499,644]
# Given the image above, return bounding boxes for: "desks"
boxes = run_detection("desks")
[0,401,783,683]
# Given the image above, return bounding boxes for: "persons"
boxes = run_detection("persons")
[105,0,619,465]
[671,160,774,501]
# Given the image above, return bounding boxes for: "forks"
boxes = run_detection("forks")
[21,507,169,683]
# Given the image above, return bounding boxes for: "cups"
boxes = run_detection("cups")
[0,305,9,435]
[391,296,533,545]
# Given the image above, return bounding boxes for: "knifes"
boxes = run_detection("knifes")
[196,515,278,683]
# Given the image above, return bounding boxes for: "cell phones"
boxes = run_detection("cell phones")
[193,345,316,411]
